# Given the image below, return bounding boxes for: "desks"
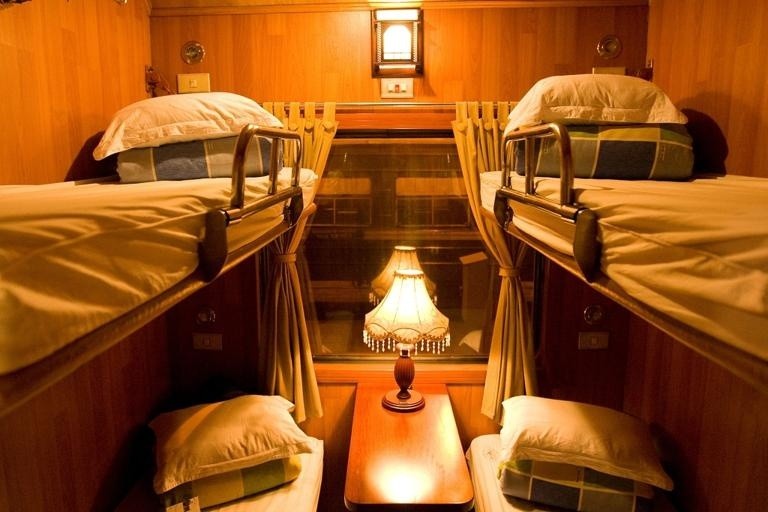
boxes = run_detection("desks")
[344,382,475,512]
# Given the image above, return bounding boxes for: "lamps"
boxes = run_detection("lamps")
[371,8,424,99]
[368,245,438,307]
[362,270,451,412]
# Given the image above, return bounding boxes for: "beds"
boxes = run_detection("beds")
[0,124,324,512]
[465,172,768,512]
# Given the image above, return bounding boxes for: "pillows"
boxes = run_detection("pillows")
[503,73,688,138]
[93,92,283,161]
[148,395,314,496]
[501,395,675,492]
